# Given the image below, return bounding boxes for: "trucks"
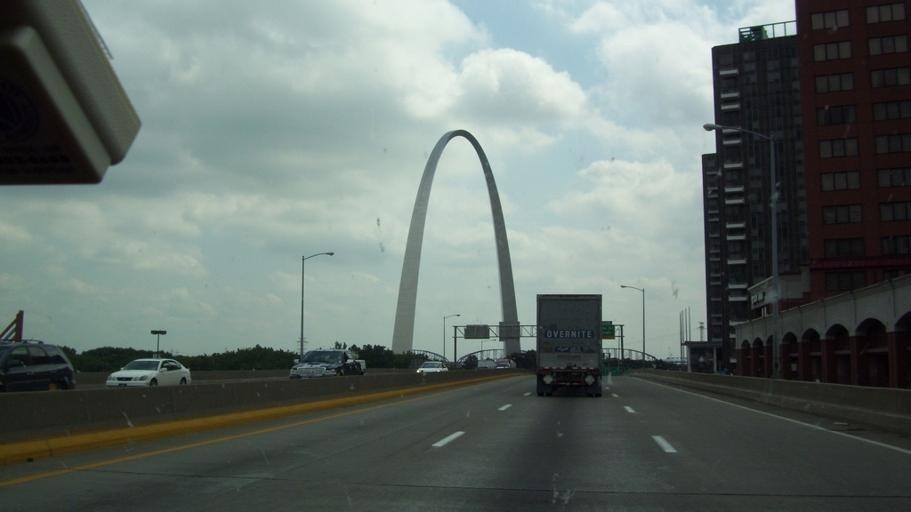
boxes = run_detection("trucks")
[494,359,517,370]
[536,293,603,397]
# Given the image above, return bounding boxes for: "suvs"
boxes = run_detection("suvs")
[0,338,77,392]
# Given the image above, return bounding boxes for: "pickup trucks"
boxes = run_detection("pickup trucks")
[289,348,367,378]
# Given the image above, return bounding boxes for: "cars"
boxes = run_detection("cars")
[106,358,192,388]
[416,360,448,375]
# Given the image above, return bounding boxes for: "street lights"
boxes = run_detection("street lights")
[443,314,460,358]
[703,122,780,311]
[150,330,167,358]
[620,284,645,363]
[300,252,335,363]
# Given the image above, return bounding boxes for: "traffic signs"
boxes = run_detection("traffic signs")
[602,320,615,339]
